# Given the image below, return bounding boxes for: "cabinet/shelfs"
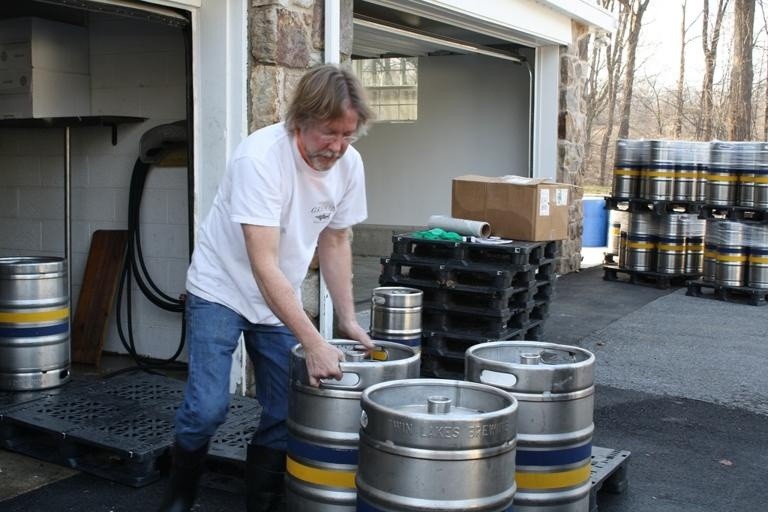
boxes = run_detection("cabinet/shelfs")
[0,9,188,121]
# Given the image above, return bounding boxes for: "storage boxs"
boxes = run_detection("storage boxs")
[452,173,573,242]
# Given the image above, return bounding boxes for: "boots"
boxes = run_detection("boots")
[245,443,287,512]
[158,441,208,512]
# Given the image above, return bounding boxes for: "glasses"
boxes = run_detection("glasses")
[321,133,359,145]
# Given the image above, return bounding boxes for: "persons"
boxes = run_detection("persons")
[158,62,379,512]
